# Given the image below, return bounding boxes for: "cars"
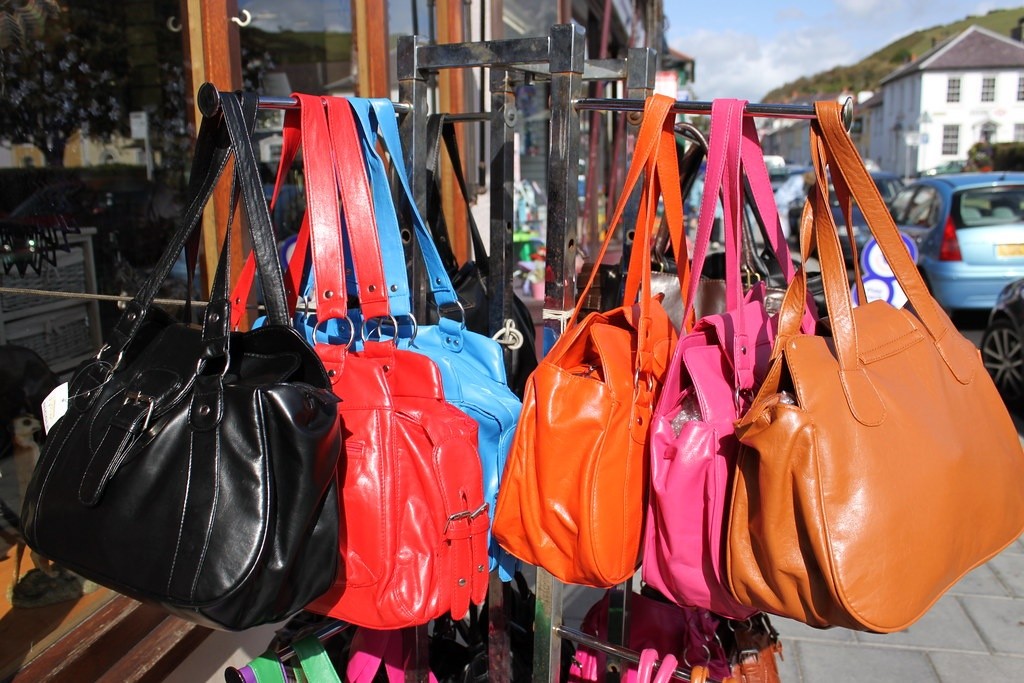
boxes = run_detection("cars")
[980,278,1024,411]
[759,155,905,267]
[889,171,1024,324]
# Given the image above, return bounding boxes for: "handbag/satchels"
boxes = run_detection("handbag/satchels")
[21,88,1024,682]
